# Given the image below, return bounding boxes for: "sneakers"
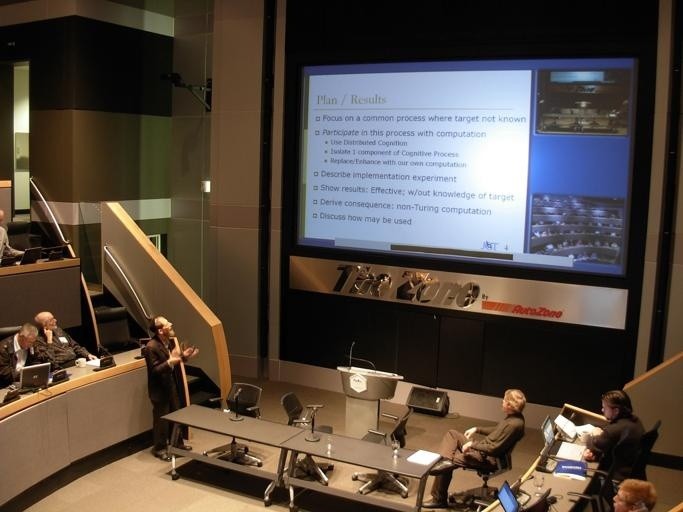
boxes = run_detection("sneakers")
[428,460,456,476]
[419,500,450,509]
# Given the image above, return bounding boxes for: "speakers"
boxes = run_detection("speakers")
[407,387,449,416]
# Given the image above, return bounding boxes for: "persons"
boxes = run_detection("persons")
[32,312,97,372]
[585,390,646,512]
[0,323,42,388]
[0,210,23,270]
[422,389,527,508]
[144,316,199,462]
[613,479,656,512]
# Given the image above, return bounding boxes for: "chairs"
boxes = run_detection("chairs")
[281,389,335,485]
[203,381,263,469]
[626,419,662,477]
[450,427,526,512]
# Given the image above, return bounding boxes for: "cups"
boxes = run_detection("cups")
[391,439,400,460]
[75,358,86,367]
[325,433,335,456]
[533,474,545,497]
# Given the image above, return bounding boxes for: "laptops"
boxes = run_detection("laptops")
[496,479,519,511]
[12,247,42,265]
[540,415,562,457]
[9,362,50,393]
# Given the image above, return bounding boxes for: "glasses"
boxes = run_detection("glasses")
[616,493,634,505]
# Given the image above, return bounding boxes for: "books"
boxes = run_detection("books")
[556,441,586,462]
[553,460,587,480]
[86,359,102,367]
[407,449,440,465]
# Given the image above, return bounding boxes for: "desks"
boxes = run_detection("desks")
[0,257,82,334]
[0,336,191,507]
[481,403,610,511]
[159,403,440,511]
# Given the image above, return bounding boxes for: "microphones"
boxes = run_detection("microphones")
[513,475,534,490]
[349,342,356,369]
[233,387,242,399]
[310,407,318,418]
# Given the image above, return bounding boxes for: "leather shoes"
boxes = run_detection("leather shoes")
[153,451,172,460]
[175,443,193,450]
